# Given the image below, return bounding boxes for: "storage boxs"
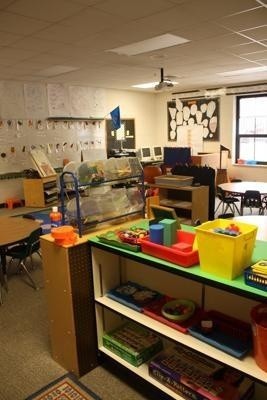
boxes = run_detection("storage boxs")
[193,220,258,280]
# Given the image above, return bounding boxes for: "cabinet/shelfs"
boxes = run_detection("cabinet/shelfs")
[88,218,267,400]
[23,176,58,207]
[60,170,210,237]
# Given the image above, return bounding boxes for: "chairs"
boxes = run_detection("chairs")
[214,179,267,216]
[5,227,43,291]
[23,215,35,221]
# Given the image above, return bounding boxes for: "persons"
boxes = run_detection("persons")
[204,366,245,400]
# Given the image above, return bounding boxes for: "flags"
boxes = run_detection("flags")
[109,106,121,131]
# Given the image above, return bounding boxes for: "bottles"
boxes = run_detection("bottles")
[49,206,62,227]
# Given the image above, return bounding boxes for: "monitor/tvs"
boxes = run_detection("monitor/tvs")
[138,147,152,160]
[152,146,164,161]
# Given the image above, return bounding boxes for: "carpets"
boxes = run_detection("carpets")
[24,372,101,400]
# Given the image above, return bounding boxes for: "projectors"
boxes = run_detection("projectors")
[155,82,173,91]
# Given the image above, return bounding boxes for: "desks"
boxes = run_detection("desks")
[0,216,41,292]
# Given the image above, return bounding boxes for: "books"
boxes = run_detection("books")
[27,152,44,178]
[30,149,57,178]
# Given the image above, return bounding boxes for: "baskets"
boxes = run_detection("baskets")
[243,265,267,293]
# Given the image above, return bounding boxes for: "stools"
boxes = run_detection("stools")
[5,198,23,209]
